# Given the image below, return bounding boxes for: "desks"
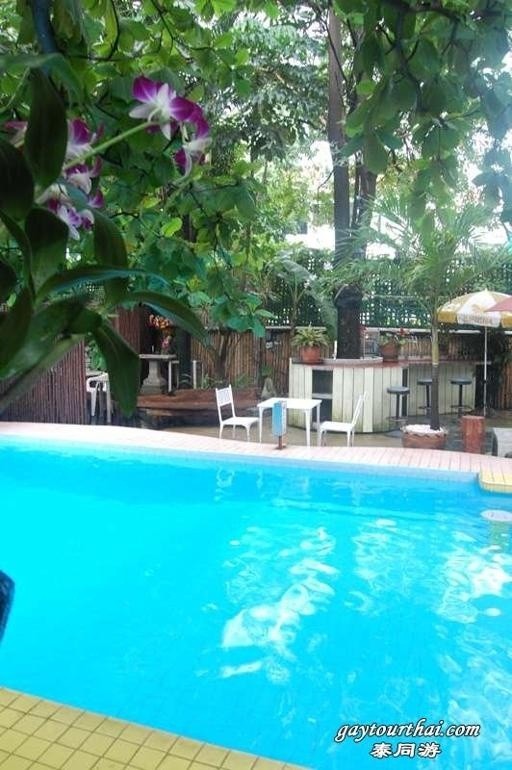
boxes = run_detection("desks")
[252,395,324,448]
[136,350,178,395]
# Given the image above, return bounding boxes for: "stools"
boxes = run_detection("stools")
[449,377,474,419]
[417,374,433,425]
[384,384,413,440]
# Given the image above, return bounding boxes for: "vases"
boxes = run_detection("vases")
[380,342,400,364]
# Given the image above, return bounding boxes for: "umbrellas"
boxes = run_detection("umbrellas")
[436,287,512,418]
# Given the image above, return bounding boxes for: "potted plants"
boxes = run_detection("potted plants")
[288,321,333,366]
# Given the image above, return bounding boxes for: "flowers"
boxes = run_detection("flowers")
[374,327,414,349]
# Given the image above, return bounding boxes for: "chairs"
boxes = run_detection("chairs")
[318,392,365,449]
[82,369,113,425]
[211,382,258,442]
